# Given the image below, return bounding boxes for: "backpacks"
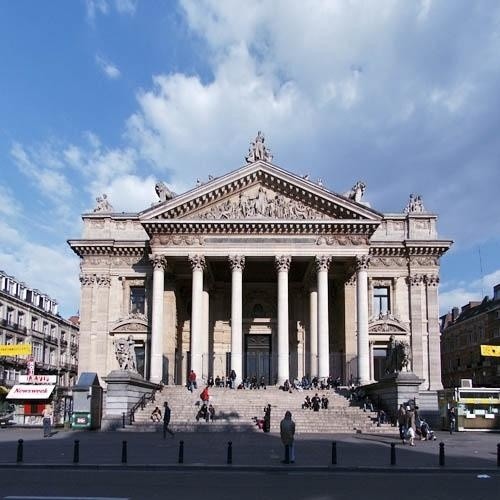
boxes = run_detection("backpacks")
[200,393,204,400]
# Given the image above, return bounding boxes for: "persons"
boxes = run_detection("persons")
[255,131,266,160]
[394,384,437,446]
[197,403,209,419]
[188,369,199,392]
[209,367,365,395]
[202,386,210,403]
[208,405,216,419]
[161,401,175,441]
[263,404,271,432]
[280,411,296,463]
[42,404,53,437]
[321,395,328,409]
[447,407,456,435]
[350,395,394,427]
[302,396,312,410]
[201,185,328,222]
[311,393,321,411]
[150,406,162,423]
[408,194,425,213]
